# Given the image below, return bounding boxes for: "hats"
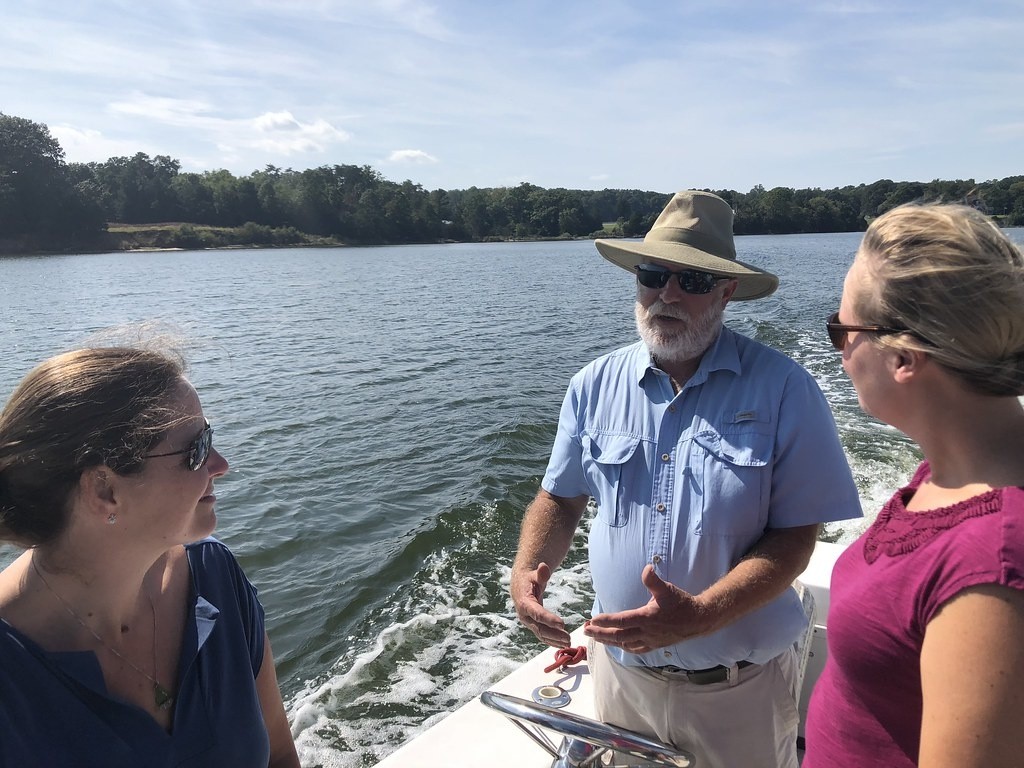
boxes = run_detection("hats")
[594,191,779,302]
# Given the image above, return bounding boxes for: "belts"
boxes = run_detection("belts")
[643,660,753,685]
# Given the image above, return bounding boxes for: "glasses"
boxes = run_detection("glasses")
[119,415,214,471]
[634,263,733,294]
[826,311,938,350]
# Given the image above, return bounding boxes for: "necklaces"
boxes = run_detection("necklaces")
[31,546,172,709]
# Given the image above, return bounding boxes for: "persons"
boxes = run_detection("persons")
[805,201,1024,768]
[511,191,862,767]
[0,347,300,768]
